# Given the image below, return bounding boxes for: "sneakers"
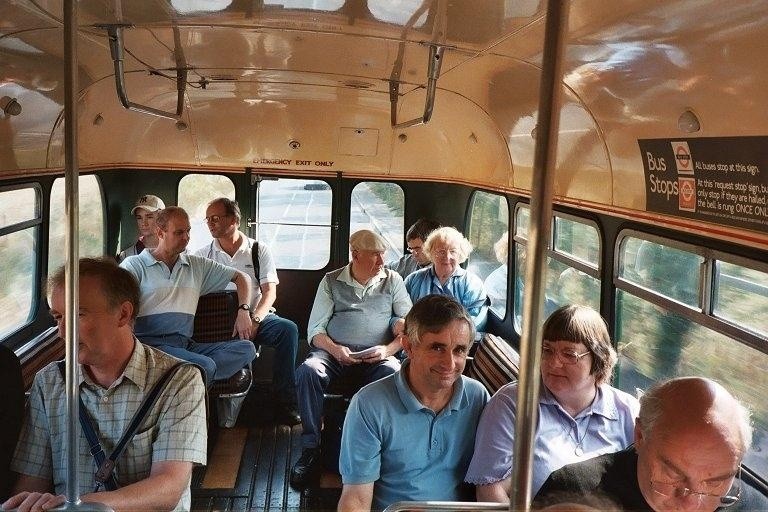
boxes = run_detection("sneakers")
[266,407,300,423]
[290,449,318,483]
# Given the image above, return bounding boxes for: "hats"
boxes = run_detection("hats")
[406,247,423,253]
[131,195,165,214]
[348,230,390,251]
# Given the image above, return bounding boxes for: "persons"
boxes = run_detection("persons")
[530,377,767,512]
[114,194,165,267]
[292,230,412,490]
[0,258,209,512]
[462,304,641,505]
[338,294,494,511]
[116,207,256,390]
[387,227,487,334]
[485,228,550,322]
[385,218,439,282]
[195,197,302,428]
[529,488,622,512]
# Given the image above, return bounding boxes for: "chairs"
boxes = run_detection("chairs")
[12,325,67,395]
[467,333,521,397]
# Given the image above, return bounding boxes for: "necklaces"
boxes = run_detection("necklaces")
[556,411,590,456]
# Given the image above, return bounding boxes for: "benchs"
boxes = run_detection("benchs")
[319,376,389,447]
[270,284,319,367]
[189,290,255,446]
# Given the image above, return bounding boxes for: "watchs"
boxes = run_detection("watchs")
[251,317,261,323]
[238,303,250,310]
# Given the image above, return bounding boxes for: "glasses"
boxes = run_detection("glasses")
[203,215,228,222]
[650,470,741,506]
[540,346,591,365]
[432,248,460,260]
